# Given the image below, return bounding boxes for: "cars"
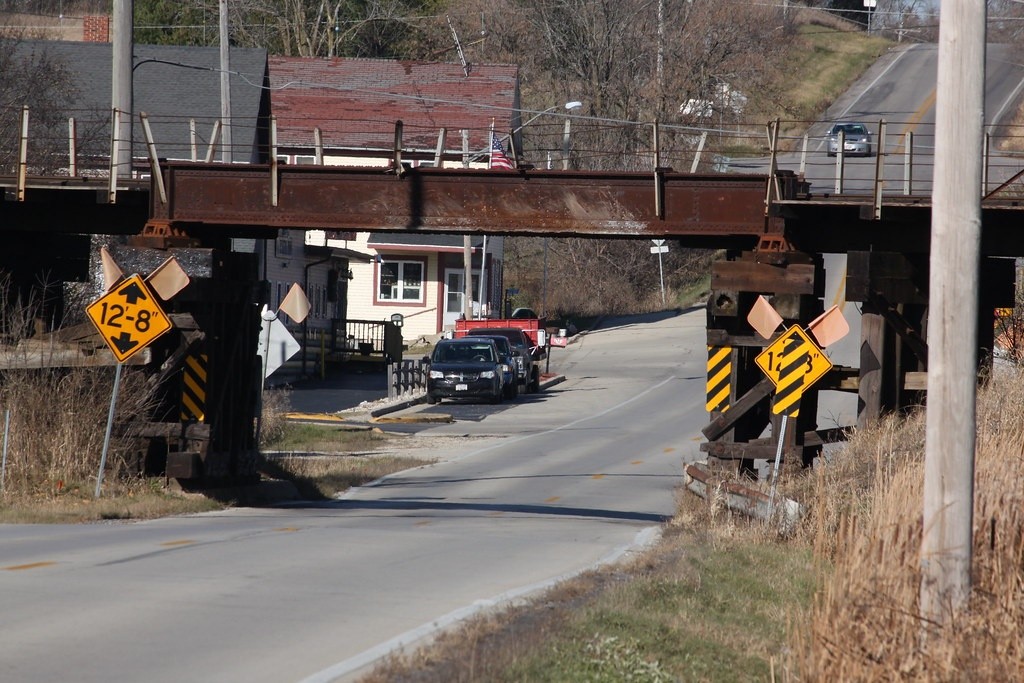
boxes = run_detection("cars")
[461,335,519,400]
[466,328,540,396]
[826,122,875,157]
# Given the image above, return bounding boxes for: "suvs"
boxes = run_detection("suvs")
[422,338,508,404]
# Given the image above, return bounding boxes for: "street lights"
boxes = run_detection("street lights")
[463,102,584,321]
